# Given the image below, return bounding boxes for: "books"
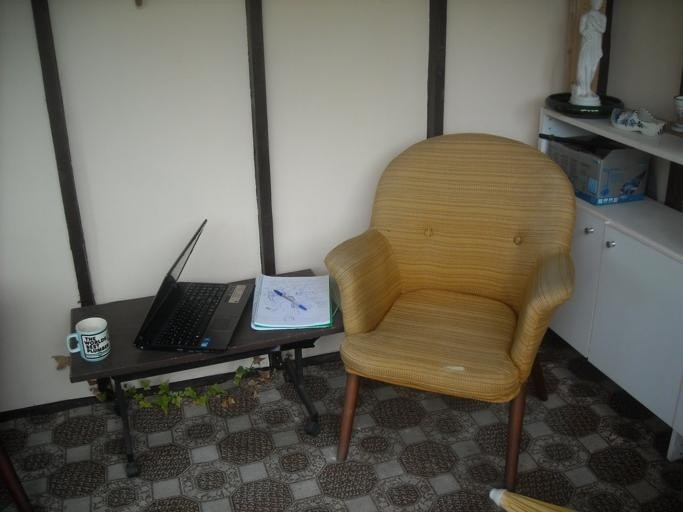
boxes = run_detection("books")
[251,273,335,331]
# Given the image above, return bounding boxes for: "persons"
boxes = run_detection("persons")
[572,1,609,98]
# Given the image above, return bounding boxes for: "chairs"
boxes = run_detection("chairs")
[322,132,578,493]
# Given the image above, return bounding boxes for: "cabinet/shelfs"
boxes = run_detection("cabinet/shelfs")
[538,101,683,464]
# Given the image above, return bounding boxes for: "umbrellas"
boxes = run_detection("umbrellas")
[488,487,574,511]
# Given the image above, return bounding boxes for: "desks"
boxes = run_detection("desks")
[68,265,343,477]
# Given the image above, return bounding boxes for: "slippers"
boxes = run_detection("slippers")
[610,107,666,137]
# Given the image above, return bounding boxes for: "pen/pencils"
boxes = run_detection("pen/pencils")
[274,289,307,310]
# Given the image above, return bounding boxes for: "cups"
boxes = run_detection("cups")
[66,317,111,361]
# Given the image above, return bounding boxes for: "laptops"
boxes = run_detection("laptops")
[134,219,254,352]
